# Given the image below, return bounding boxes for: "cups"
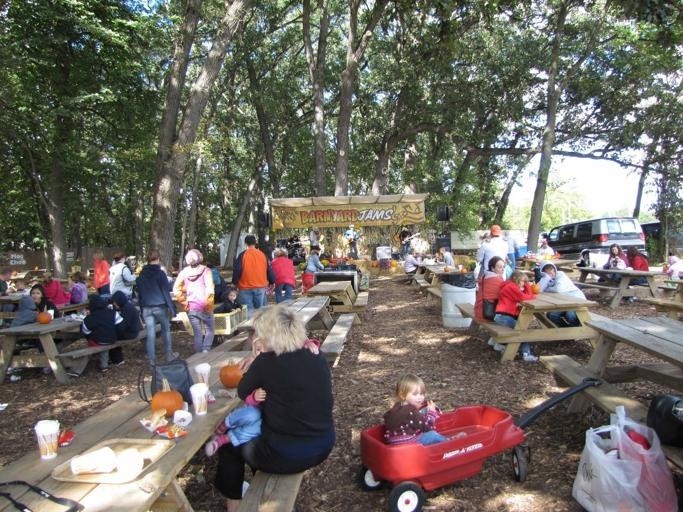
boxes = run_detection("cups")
[189,383,207,415]
[35,421,59,458]
[195,363,209,385]
[48,309,55,321]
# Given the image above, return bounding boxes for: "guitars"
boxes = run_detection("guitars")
[286,241,302,249]
[400,233,422,245]
[347,232,364,244]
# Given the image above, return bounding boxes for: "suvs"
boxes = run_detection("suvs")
[540,231,558,241]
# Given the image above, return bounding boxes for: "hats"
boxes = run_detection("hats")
[490,224,501,236]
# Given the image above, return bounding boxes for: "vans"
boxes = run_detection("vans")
[543,217,650,265]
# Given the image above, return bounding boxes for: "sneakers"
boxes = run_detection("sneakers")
[203,434,220,456]
[522,352,538,362]
[163,350,181,362]
[211,430,220,439]
[100,367,114,375]
[487,336,500,346]
[107,359,126,368]
[493,343,507,352]
[65,370,79,378]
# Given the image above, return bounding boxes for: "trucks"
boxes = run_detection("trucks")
[439,230,491,256]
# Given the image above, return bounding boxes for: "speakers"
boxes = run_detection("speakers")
[436,205,449,222]
[259,212,271,228]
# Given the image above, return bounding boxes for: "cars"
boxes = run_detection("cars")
[17,269,46,282]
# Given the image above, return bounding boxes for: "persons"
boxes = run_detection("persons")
[302,250,325,293]
[474,224,649,361]
[383,373,468,446]
[270,247,297,304]
[400,225,455,274]
[344,224,360,257]
[205,334,320,457]
[213,307,335,512]
[233,234,275,320]
[0,248,242,376]
[309,226,322,254]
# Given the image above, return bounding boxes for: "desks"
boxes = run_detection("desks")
[575,266,667,310]
[523,258,580,271]
[313,271,359,293]
[307,281,361,325]
[415,262,460,301]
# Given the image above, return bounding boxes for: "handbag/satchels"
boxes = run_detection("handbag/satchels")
[645,392,683,447]
[481,298,496,320]
[148,359,195,405]
[123,280,132,287]
